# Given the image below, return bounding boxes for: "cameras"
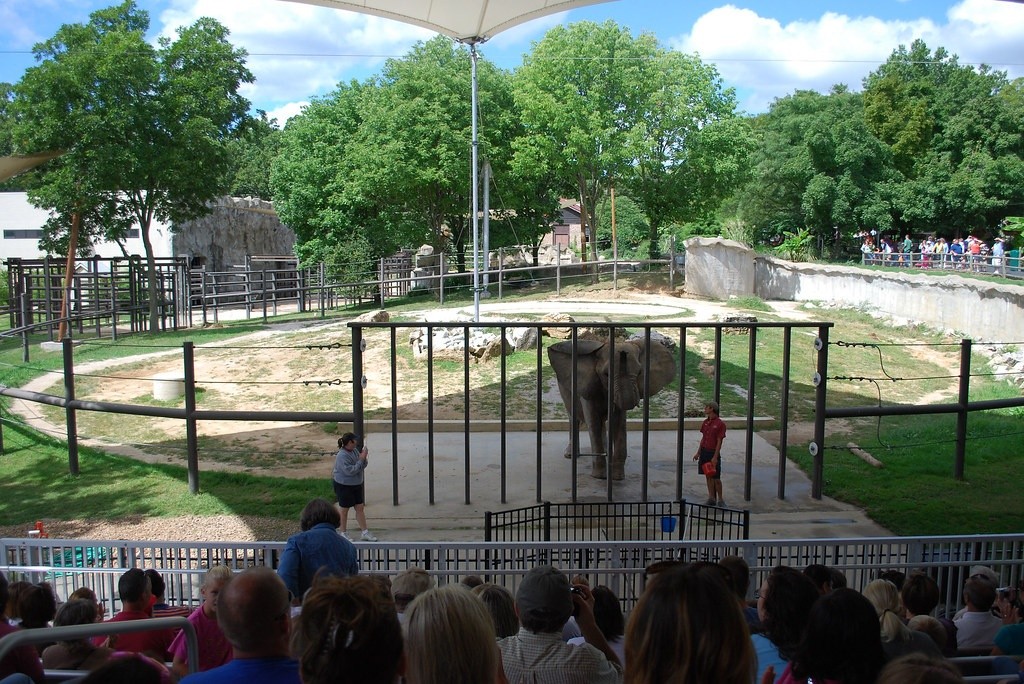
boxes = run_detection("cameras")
[570,585,586,596]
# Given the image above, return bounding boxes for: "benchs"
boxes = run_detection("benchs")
[946,644,1022,684]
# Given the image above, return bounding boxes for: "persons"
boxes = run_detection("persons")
[0,569,194,684]
[498,565,626,684]
[953,566,1024,684]
[169,568,405,684]
[863,570,965,684]
[626,556,887,683]
[278,499,357,604]
[392,567,519,683]
[692,402,726,507]
[333,432,378,541]
[902,572,957,658]
[860,229,1005,274]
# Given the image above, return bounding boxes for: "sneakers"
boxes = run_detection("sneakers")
[360,532,377,541]
[340,532,354,542]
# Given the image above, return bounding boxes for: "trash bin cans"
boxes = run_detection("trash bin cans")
[1009,250,1019,272]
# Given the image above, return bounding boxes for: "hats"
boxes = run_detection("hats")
[704,401,719,410]
[970,565,999,586]
[517,565,572,622]
[391,566,430,605]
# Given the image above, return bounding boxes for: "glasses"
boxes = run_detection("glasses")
[754,590,767,601]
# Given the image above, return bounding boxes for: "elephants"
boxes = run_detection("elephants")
[547,339,676,480]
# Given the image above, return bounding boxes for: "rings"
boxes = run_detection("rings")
[1004,614,1006,617]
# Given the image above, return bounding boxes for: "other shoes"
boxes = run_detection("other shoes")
[702,499,716,508]
[718,501,728,508]
